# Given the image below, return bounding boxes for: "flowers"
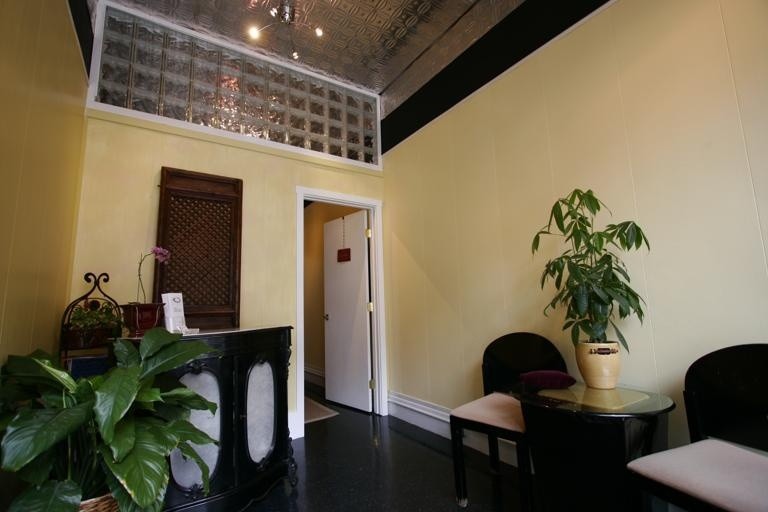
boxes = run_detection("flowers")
[135,246,170,300]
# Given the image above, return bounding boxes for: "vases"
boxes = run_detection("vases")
[121,299,166,335]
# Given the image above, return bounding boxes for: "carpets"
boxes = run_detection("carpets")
[304,398,339,426]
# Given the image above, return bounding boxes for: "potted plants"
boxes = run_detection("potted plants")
[5,328,224,512]
[526,187,652,391]
[61,272,120,348]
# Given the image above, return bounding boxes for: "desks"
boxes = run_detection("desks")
[520,388,676,512]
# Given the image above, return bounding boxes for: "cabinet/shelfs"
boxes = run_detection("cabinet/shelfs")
[68,323,300,512]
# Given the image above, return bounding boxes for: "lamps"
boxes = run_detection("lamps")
[239,0,328,62]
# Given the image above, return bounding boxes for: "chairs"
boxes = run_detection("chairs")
[626,339,768,512]
[445,332,569,507]
